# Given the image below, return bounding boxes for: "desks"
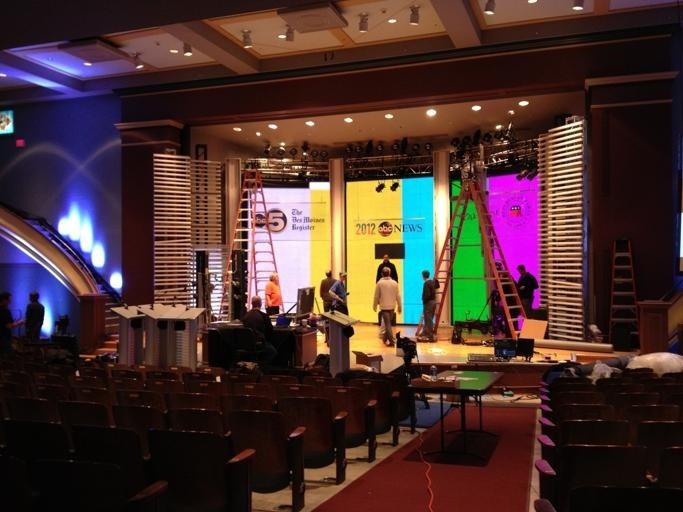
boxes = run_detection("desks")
[200,326,317,368]
[406,369,504,453]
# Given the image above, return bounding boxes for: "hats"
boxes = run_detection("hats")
[339,272,347,277]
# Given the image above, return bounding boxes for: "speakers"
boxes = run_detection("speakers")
[517,338,534,358]
[495,338,517,357]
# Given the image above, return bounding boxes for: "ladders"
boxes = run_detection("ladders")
[609,237,639,343]
[217,169,285,322]
[415,178,528,339]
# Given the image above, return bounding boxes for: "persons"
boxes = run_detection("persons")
[319,271,349,345]
[264,271,283,314]
[372,255,402,347]
[25,291,44,336]
[241,296,277,360]
[514,265,538,318]
[0,292,25,339]
[422,271,440,341]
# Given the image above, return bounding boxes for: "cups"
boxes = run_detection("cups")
[569,352,576,362]
[464,311,471,322]
[301,319,307,330]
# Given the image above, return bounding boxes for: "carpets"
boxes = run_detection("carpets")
[398,399,452,429]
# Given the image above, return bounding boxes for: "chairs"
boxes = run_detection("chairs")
[0,353,416,512]
[221,328,256,368]
[534,366,683,512]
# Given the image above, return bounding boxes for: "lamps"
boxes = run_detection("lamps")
[409,6,419,25]
[572,0,584,11]
[263,128,538,193]
[241,29,252,49]
[285,24,296,41]
[483,0,495,16]
[183,42,192,57]
[131,52,144,70]
[358,12,368,32]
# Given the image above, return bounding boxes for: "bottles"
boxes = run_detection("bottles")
[462,327,468,341]
[430,363,437,383]
[53,324,59,336]
[11,319,25,328]
